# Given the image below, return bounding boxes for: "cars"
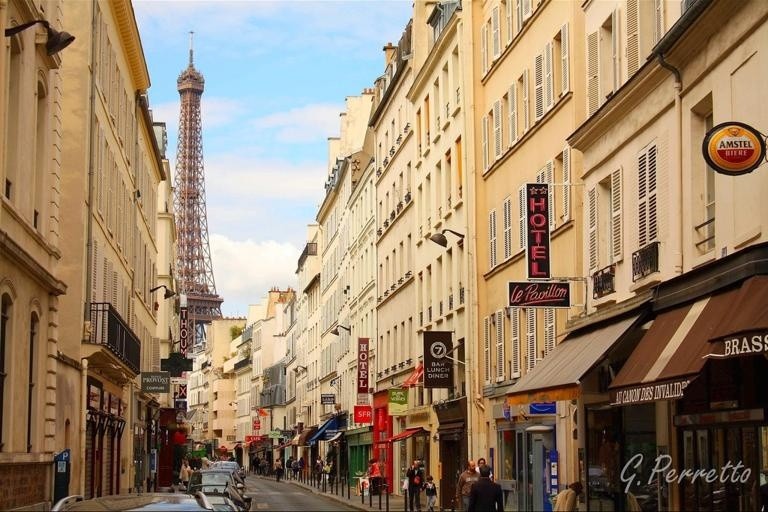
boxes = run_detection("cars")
[52,462,252,512]
[589,465,611,497]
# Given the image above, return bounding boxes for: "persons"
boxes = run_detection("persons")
[467,464,503,512]
[549,480,583,512]
[418,475,437,512]
[251,455,335,487]
[405,460,423,512]
[455,460,479,512]
[364,459,382,496]
[474,458,493,482]
[177,455,194,489]
